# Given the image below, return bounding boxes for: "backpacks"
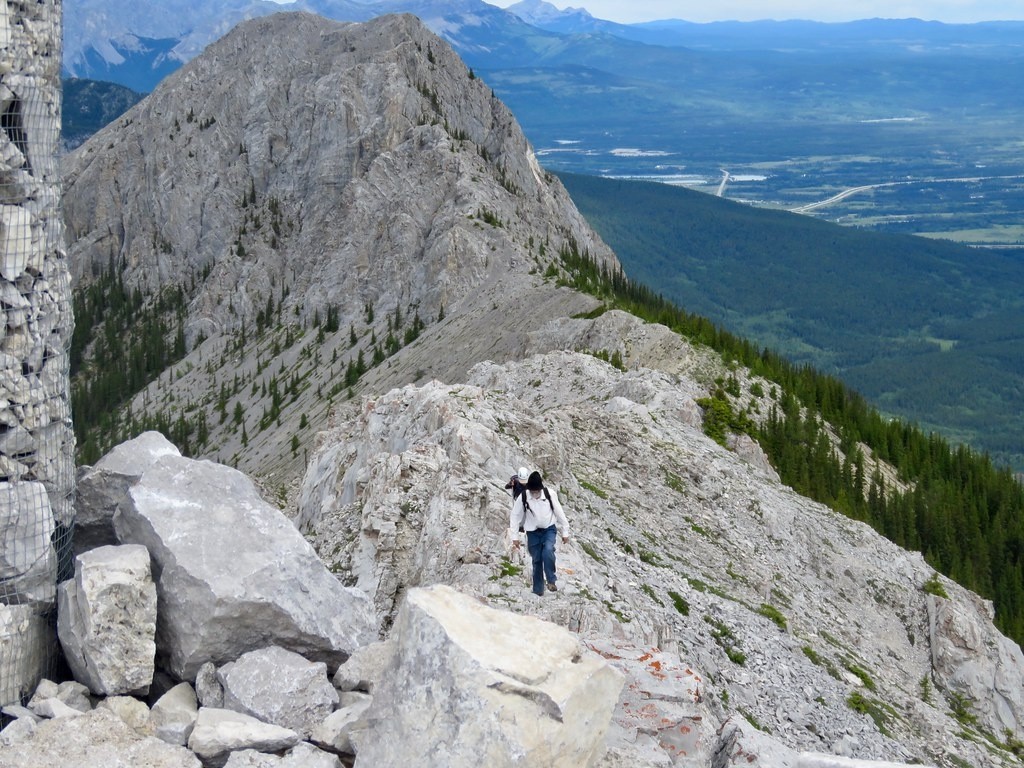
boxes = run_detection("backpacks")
[506,475,554,512]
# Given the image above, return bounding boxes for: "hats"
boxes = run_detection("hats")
[526,471,544,490]
[518,467,529,484]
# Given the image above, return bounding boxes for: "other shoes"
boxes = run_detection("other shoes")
[548,582,557,592]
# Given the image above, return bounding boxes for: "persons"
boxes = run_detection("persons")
[509,470,570,597]
[505,467,533,567]
